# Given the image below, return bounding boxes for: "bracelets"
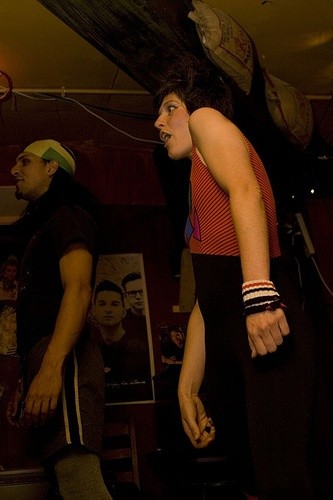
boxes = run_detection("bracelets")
[242,279,279,316]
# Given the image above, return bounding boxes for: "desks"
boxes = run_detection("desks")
[103,417,140,491]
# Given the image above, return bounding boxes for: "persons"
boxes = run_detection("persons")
[95,271,186,385]
[154,56,292,500]
[10,139,114,500]
[0,260,18,300]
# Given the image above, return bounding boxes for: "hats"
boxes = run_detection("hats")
[23,139,76,176]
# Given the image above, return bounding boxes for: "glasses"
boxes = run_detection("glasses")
[124,289,144,298]
